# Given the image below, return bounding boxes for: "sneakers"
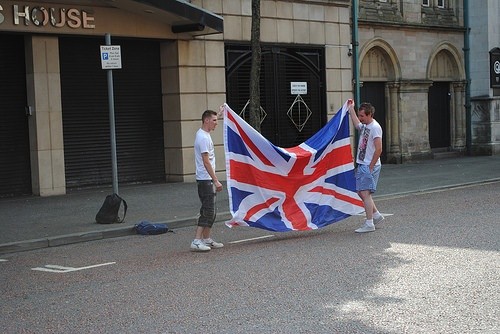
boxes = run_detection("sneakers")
[190,240,211,251]
[355,223,375,232]
[202,238,223,248]
[373,215,385,225]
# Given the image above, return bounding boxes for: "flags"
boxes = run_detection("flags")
[224,101,366,233]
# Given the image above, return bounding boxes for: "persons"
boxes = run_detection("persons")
[347,100,384,233]
[191,105,224,251]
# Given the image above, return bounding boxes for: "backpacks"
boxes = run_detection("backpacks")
[135,221,168,235]
[96,194,127,224]
